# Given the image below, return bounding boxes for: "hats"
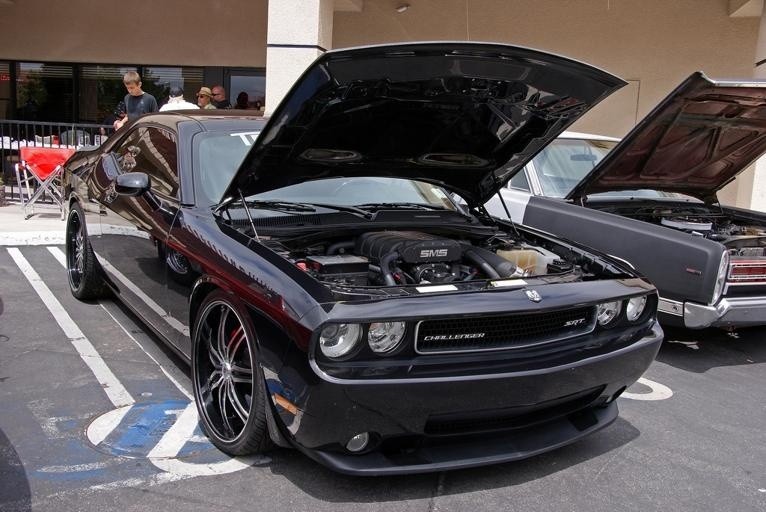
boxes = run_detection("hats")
[169,86,184,96]
[196,87,213,98]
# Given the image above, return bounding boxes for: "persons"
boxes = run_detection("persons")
[98,71,254,132]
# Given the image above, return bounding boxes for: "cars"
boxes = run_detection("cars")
[57,41,665,481]
[471,70,766,347]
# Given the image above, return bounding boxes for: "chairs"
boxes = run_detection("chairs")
[14,146,76,221]
[35,129,108,147]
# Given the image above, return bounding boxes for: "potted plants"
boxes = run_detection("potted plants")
[0,180,6,206]
